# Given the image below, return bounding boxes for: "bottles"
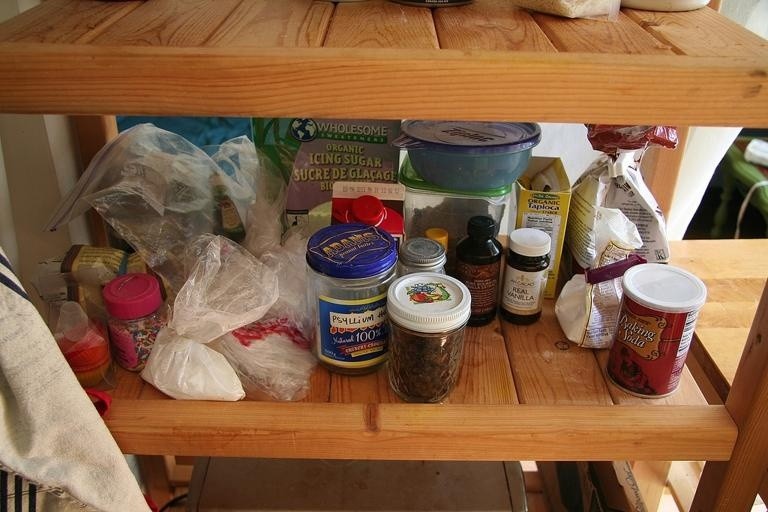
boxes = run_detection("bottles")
[102,271,172,371]
[402,227,450,276]
[500,225,553,325]
[454,215,505,329]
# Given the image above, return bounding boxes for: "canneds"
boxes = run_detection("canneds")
[606,262,707,399]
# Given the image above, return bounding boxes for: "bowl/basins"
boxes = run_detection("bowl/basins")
[390,121,543,190]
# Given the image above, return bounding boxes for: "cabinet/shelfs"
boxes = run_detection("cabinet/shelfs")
[2,0,768,511]
